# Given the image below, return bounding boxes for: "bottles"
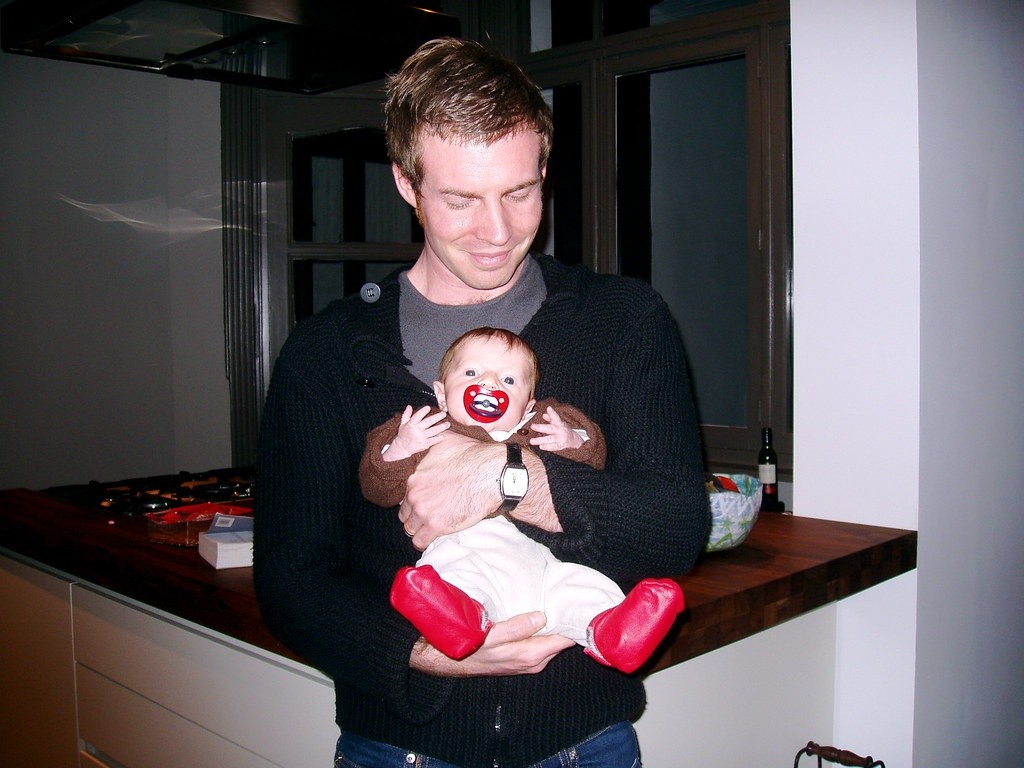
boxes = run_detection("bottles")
[756,427,777,512]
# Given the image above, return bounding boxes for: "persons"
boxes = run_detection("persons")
[360,326,685,674]
[253,38,713,768]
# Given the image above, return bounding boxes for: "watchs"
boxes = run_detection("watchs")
[500,442,528,511]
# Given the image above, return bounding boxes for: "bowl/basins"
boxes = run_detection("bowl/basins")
[701,473,762,551]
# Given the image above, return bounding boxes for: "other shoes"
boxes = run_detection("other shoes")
[389,563,489,661]
[589,577,685,674]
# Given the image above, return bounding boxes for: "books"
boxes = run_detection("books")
[198,512,253,569]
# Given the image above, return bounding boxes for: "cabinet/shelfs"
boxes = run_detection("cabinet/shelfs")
[0,553,340,768]
